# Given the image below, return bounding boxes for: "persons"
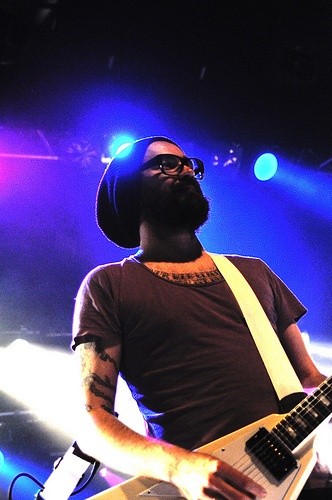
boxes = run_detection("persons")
[74,136,332,496]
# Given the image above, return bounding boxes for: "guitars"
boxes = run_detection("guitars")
[87,376,332,500]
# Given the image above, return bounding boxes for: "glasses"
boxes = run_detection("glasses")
[141,154,205,180]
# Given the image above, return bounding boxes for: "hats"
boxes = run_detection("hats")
[96,136,188,250]
